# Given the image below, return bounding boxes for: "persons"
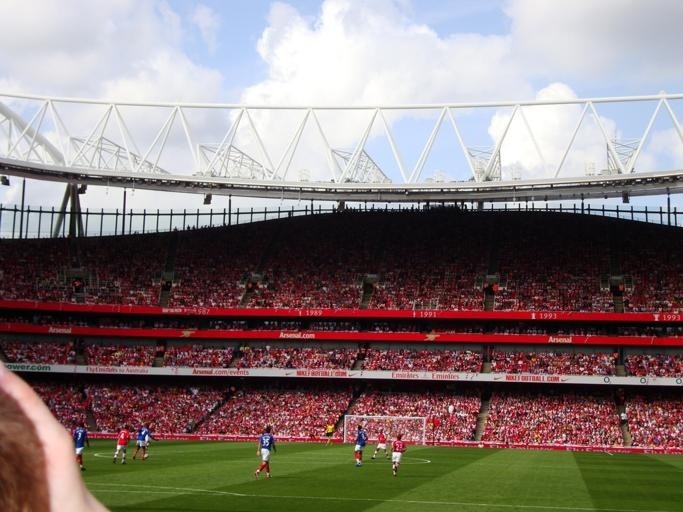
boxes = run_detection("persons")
[389,434,407,478]
[368,426,389,461]
[111,423,131,463]
[132,421,150,459]
[351,424,367,467]
[253,426,276,481]
[72,422,89,469]
[0,207,683,451]
